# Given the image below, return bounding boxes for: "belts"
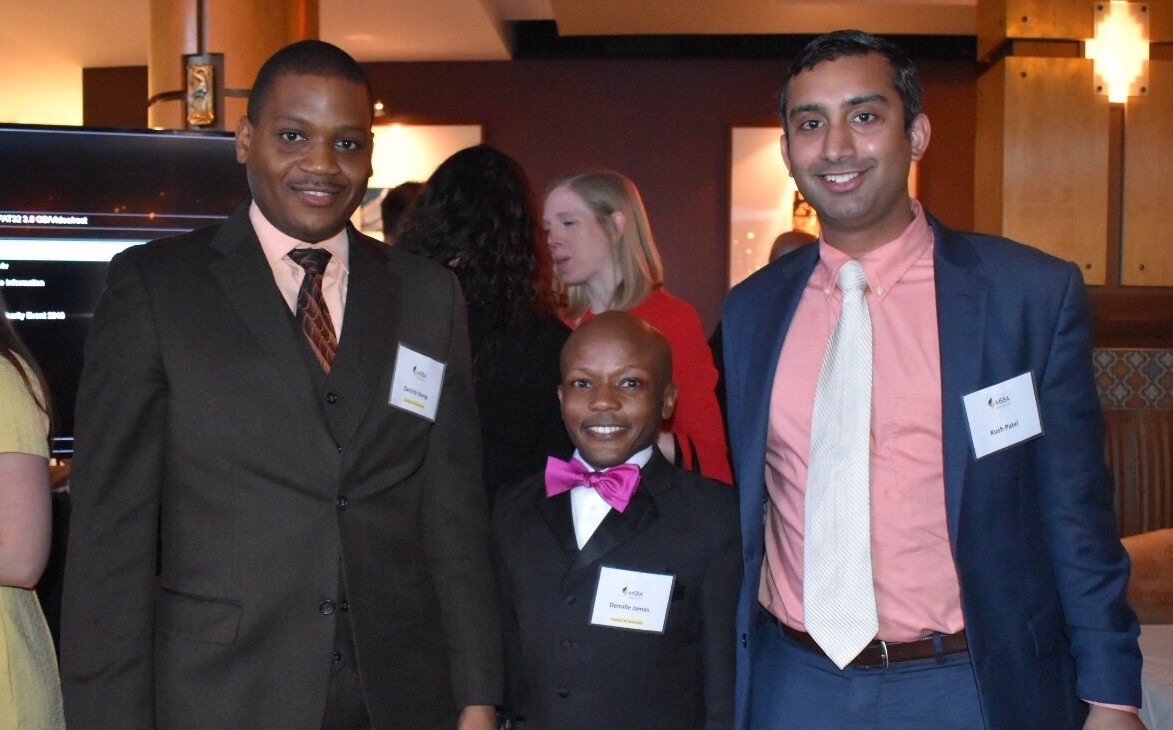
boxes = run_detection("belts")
[772,621,967,665]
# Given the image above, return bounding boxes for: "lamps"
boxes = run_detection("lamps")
[1079,2,1149,103]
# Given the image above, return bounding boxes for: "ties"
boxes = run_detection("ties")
[804,260,879,672]
[289,249,337,375]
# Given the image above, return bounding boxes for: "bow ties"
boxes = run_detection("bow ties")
[545,454,642,513]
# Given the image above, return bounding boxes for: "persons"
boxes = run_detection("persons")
[710,231,819,476]
[541,170,732,486]
[59,39,500,730]
[496,309,744,730]
[390,144,576,518]
[0,280,66,730]
[381,182,430,247]
[721,29,1148,730]
